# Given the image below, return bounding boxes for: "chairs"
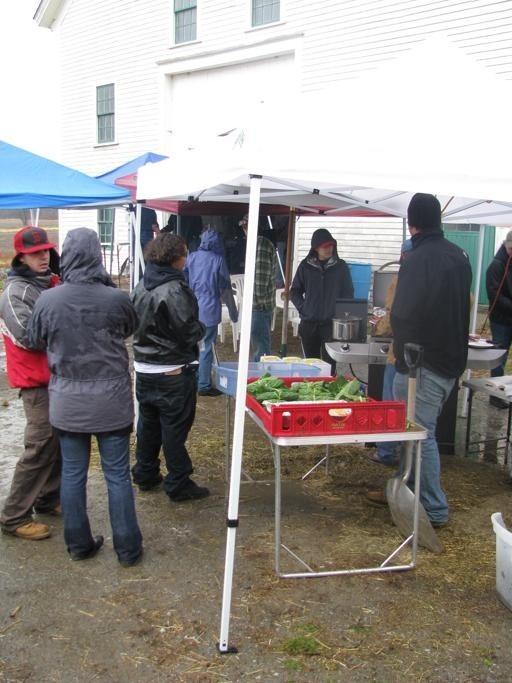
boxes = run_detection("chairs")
[215,273,302,351]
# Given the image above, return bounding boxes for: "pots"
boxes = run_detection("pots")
[332,311,362,340]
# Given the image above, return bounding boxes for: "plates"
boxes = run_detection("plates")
[468,342,494,348]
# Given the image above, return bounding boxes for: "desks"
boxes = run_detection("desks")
[461,375,512,464]
[221,391,428,579]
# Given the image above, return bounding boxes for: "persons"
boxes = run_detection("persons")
[29,226,143,567]
[290,227,354,378]
[183,229,238,397]
[369,238,414,464]
[239,213,284,361]
[129,231,209,501]
[151,195,204,247]
[1,225,64,540]
[364,192,472,527]
[131,198,159,278]
[485,229,512,409]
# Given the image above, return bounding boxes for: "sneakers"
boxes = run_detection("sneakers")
[489,397,510,409]
[138,473,164,491]
[119,546,144,568]
[11,519,51,541]
[198,387,223,398]
[168,480,211,503]
[368,451,381,464]
[86,535,105,559]
[365,490,388,505]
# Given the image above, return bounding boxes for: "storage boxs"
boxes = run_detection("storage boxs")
[489,510,512,613]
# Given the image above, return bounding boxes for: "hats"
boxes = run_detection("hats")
[401,239,414,253]
[407,192,442,230]
[12,225,57,255]
